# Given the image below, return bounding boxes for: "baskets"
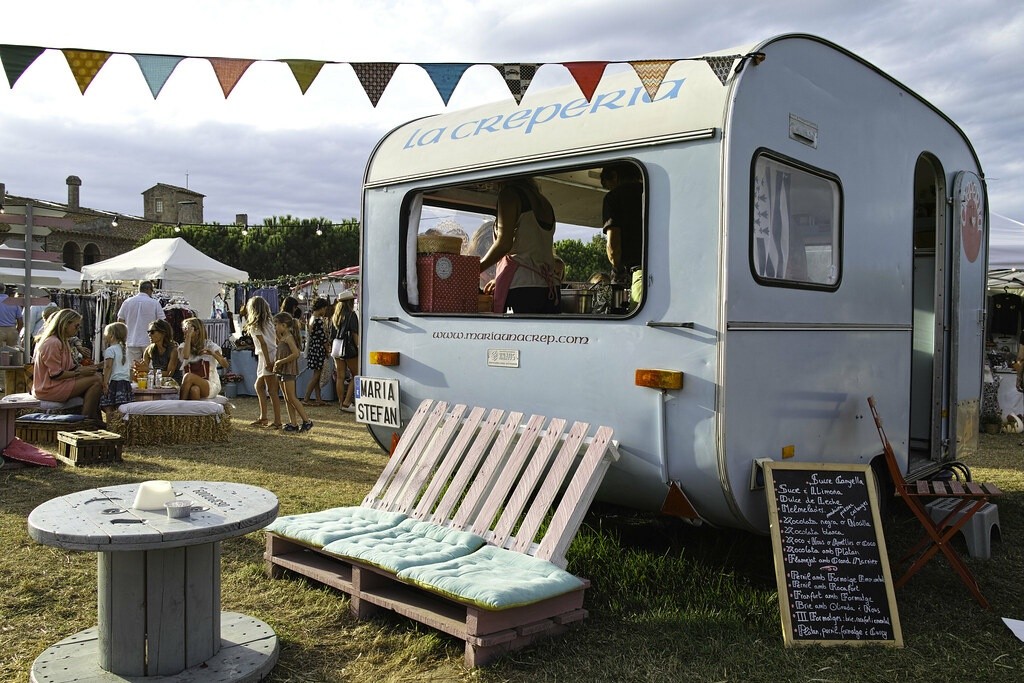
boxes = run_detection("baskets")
[417,236,463,255]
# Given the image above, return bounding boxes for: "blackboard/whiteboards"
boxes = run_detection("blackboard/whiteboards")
[762,461,905,649]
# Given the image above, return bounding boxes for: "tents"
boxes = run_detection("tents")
[0,243,82,290]
[80,236,249,317]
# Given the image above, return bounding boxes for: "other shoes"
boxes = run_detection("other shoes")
[302,402,316,407]
[340,404,356,412]
[315,401,332,406]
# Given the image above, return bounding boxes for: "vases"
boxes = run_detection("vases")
[226,382,237,398]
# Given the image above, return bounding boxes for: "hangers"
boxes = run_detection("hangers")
[112,291,189,307]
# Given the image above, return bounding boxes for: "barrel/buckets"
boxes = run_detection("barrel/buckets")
[605,284,623,308]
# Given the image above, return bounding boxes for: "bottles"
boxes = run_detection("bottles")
[147,369,155,389]
[155,368,162,389]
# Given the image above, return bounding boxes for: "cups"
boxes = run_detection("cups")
[137,378,147,390]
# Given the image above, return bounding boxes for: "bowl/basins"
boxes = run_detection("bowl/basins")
[132,480,175,510]
[163,499,195,519]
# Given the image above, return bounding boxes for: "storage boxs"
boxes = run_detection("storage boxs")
[417,254,480,312]
[57,429,123,467]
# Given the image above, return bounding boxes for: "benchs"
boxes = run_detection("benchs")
[2,393,84,419]
[107,401,226,446]
[200,395,233,434]
[264,399,620,669]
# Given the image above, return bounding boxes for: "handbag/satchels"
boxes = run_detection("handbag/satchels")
[221,340,232,359]
[320,357,334,388]
[184,359,209,380]
[331,335,358,358]
[221,302,235,333]
[19,328,35,351]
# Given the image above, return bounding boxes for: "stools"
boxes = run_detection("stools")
[926,498,1003,562]
[15,413,94,445]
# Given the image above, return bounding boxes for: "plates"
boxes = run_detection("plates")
[161,386,180,389]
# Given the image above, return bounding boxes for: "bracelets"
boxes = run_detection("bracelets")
[74,370,80,377]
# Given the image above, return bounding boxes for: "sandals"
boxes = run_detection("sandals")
[251,418,268,427]
[263,422,282,429]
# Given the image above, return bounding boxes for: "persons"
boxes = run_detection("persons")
[0,282,359,435]
[1016,329,1024,393]
[600,163,642,311]
[470,221,497,293]
[553,254,573,289]
[591,272,611,284]
[447,229,470,254]
[478,175,562,315]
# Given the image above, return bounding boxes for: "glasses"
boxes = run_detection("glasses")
[71,323,80,330]
[150,288,153,291]
[147,330,159,334]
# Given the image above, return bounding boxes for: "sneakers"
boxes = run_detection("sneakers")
[299,420,313,433]
[283,423,299,431]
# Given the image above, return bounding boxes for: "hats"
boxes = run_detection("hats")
[337,289,356,301]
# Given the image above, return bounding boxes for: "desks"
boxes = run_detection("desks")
[129,387,180,402]
[231,350,335,400]
[28,480,279,683]
[980,364,1024,423]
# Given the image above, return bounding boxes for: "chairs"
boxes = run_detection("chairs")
[866,395,1003,608]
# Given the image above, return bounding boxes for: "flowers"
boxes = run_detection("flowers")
[220,371,243,384]
